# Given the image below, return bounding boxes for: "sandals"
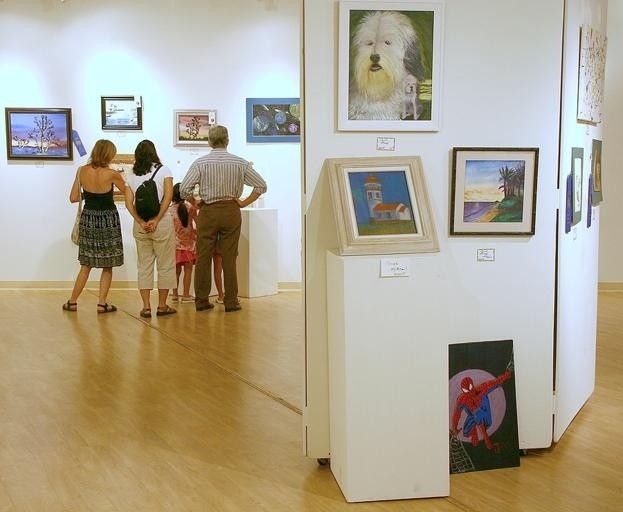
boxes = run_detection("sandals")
[137,306,153,318]
[215,294,224,303]
[156,304,178,315]
[235,297,242,303]
[97,301,118,313]
[62,299,80,312]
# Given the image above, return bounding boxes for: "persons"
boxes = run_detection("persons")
[179,124,267,311]
[62,140,125,313]
[125,139,178,317]
[214,254,241,304]
[169,183,196,303]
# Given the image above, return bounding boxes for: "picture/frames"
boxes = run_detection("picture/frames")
[326,156,440,256]
[246,97,299,142]
[572,147,583,225]
[5,107,72,161]
[451,147,539,237]
[108,154,136,201]
[336,0,445,131]
[174,108,217,147]
[101,95,142,130]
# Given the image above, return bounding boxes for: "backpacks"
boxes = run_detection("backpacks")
[135,164,166,220]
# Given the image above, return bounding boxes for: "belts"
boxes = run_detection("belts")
[212,200,236,204]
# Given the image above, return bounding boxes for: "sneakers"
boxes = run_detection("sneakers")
[195,302,214,311]
[223,305,242,313]
[182,296,197,302]
[171,294,178,303]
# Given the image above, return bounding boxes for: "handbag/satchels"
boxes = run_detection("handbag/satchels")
[70,210,82,246]
